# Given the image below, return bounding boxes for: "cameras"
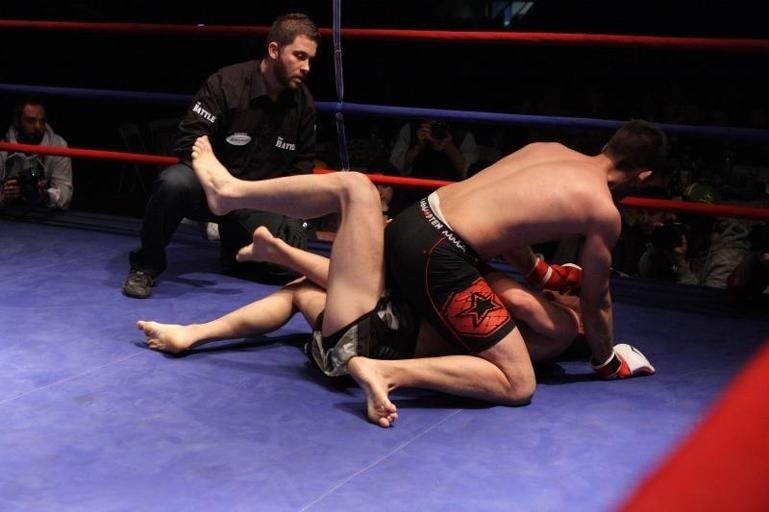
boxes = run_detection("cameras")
[17,167,40,204]
[429,122,447,140]
[650,215,693,250]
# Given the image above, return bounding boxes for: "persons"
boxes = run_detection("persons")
[122,12,319,297]
[134,134,589,388]
[0,102,73,212]
[233,119,664,428]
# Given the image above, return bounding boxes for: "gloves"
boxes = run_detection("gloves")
[592,344,656,380]
[279,217,309,252]
[526,253,584,295]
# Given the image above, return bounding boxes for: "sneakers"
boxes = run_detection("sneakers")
[124,268,153,298]
[215,222,248,268]
